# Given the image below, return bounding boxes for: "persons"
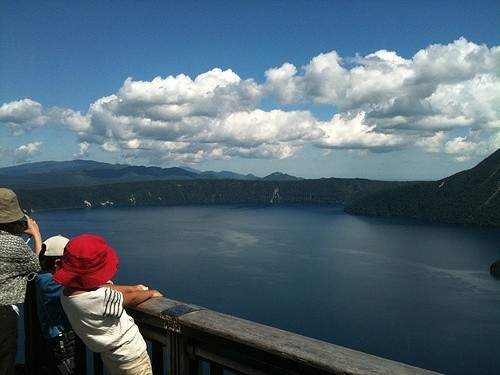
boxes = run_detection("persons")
[54,235,163,375]
[0,187,43,375]
[34,235,114,375]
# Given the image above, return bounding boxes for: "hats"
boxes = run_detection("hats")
[52,235,119,291]
[0,188,25,223]
[43,236,69,257]
